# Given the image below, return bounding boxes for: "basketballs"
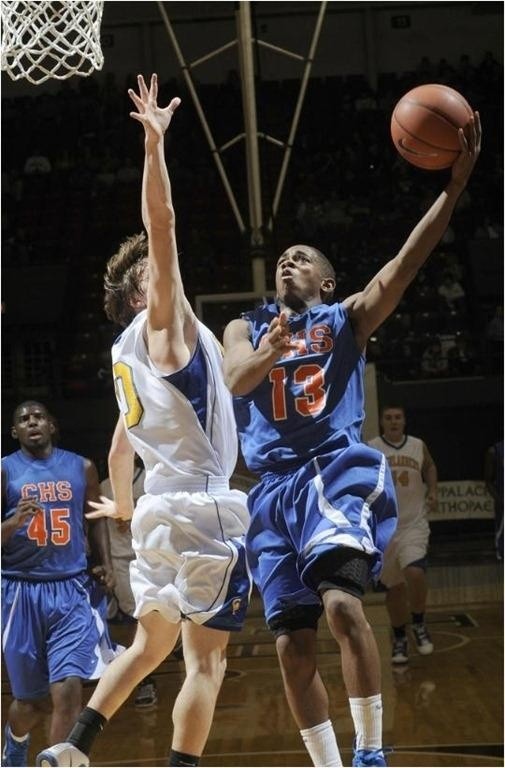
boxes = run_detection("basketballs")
[391,85,474,168]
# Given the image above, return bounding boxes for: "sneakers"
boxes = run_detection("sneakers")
[391,635,411,664]
[2,720,30,766]
[133,681,158,713]
[412,622,435,656]
[36,742,90,766]
[351,735,395,767]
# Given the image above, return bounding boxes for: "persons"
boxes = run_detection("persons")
[1,401,116,766]
[484,437,504,563]
[2,201,504,402]
[96,451,157,712]
[37,73,252,767]
[227,111,483,767]
[362,405,438,667]
